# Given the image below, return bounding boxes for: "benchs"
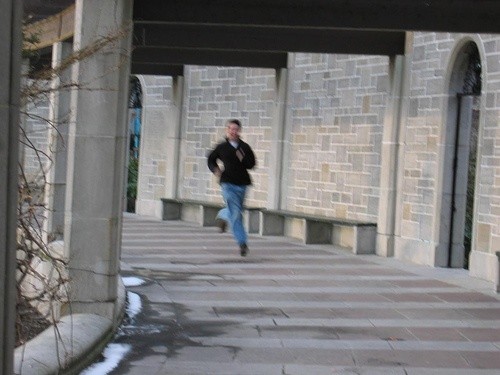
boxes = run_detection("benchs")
[258,209,378,255]
[159,198,267,235]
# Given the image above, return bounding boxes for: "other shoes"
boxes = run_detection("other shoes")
[216,217,227,233]
[239,244,249,257]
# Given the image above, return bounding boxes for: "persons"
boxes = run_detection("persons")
[207,119,257,255]
